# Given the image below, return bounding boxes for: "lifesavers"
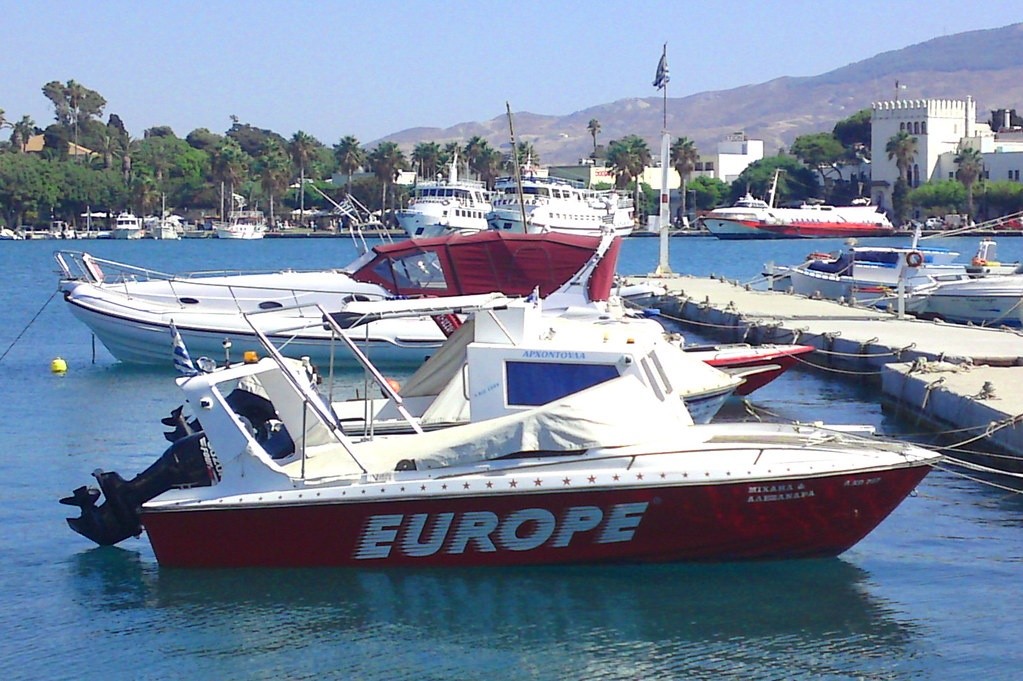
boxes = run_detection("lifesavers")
[810,253,831,258]
[907,252,922,267]
[807,256,829,260]
[442,200,449,205]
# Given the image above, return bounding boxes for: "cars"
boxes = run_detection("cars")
[897,220,926,230]
[924,218,944,231]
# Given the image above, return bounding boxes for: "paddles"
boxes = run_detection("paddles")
[322,305,507,331]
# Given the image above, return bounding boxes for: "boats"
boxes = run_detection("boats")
[486,148,635,238]
[151,191,179,239]
[0,205,113,240]
[113,209,143,240]
[52,97,817,436]
[394,150,492,240]
[699,168,897,239]
[760,225,1023,326]
[210,182,268,239]
[58,293,946,570]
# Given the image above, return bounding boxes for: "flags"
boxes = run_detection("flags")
[652,49,670,91]
